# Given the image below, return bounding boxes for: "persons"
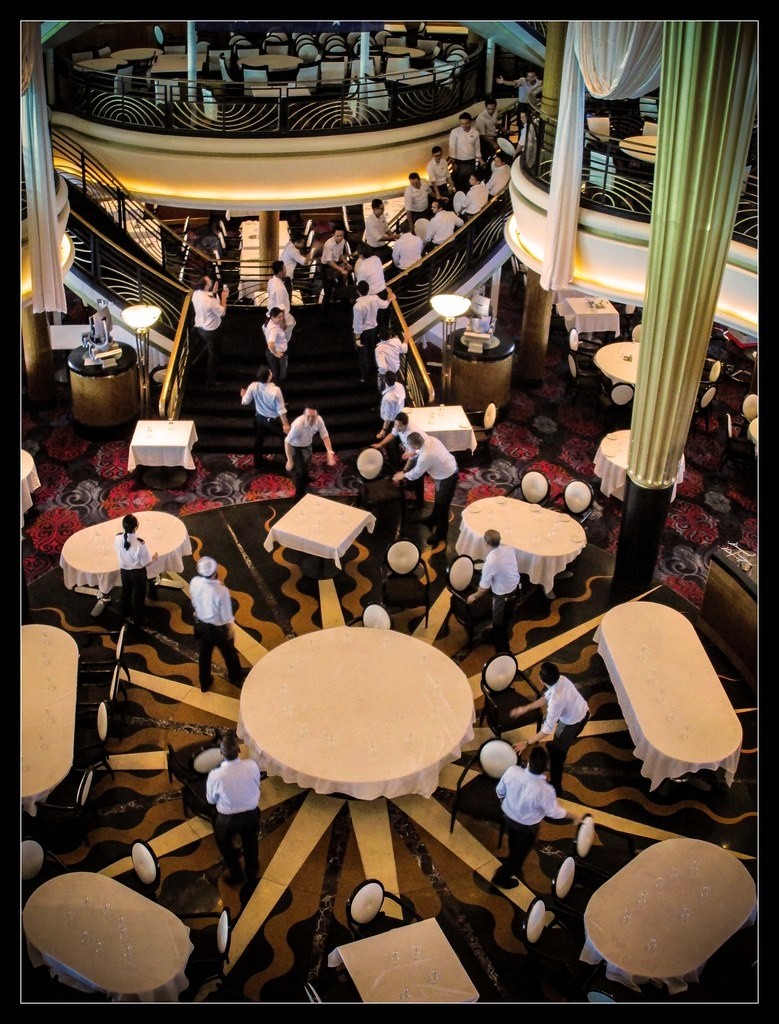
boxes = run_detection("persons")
[260,307,289,385]
[511,662,592,794]
[206,732,262,890]
[191,275,229,387]
[266,260,291,315]
[282,403,337,499]
[319,66,540,323]
[190,556,251,694]
[240,365,291,474]
[115,512,152,624]
[278,234,313,305]
[466,529,520,651]
[371,325,458,548]
[352,280,397,383]
[492,745,585,891]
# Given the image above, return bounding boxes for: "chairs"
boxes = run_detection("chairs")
[20,33,757,1005]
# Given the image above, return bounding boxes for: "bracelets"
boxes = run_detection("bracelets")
[402,468,407,473]
[382,428,387,431]
[356,338,360,340]
[327,450,335,455]
[473,594,477,598]
[409,222,412,225]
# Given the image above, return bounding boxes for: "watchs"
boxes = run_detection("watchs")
[524,740,529,746]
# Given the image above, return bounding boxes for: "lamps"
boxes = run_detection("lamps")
[122,304,161,420]
[430,294,471,403]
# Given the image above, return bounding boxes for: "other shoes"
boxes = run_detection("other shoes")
[493,878,519,890]
[202,675,214,692]
[224,871,240,889]
[427,533,447,545]
[420,515,437,525]
[238,666,251,679]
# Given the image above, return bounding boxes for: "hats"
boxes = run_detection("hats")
[197,557,216,576]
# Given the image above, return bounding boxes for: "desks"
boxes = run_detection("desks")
[593,600,742,793]
[446,327,515,413]
[456,496,587,594]
[60,511,192,594]
[619,136,657,164]
[24,872,194,1002]
[264,491,377,580]
[21,623,79,816]
[380,46,425,59]
[328,916,480,1003]
[150,52,206,71]
[595,340,642,385]
[561,298,620,351]
[593,429,686,503]
[579,837,756,995]
[20,449,41,527]
[74,57,128,73]
[237,54,304,72]
[127,420,198,489]
[67,341,138,426]
[110,48,163,60]
[235,626,476,801]
[400,405,477,456]
